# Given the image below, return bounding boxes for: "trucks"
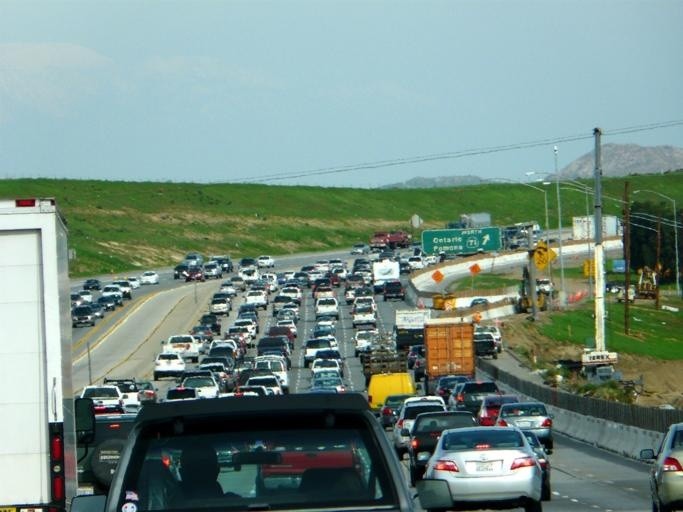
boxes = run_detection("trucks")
[1,197,78,512]
[571,214,623,240]
[422,325,476,390]
[373,261,401,293]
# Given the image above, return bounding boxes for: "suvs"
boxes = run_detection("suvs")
[78,415,179,495]
[368,231,409,250]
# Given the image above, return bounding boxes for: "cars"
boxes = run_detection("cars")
[641,420,683,512]
[382,395,415,429]
[393,403,447,460]
[193,244,382,358]
[380,251,441,274]
[408,326,503,383]
[497,403,552,451]
[534,279,555,295]
[383,280,405,300]
[77,334,352,413]
[431,376,516,425]
[70,271,159,328]
[521,431,552,502]
[403,410,476,481]
[174,253,233,282]
[500,221,544,249]
[423,423,539,510]
[72,388,452,511]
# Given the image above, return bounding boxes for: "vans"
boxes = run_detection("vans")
[403,397,446,412]
[368,373,417,415]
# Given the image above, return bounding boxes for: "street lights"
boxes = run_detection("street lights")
[525,170,592,298]
[479,176,553,293]
[633,190,679,292]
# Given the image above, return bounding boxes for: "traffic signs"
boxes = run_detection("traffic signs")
[422,227,502,256]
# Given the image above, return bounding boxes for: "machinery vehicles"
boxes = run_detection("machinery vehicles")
[636,266,658,300]
[360,349,408,386]
